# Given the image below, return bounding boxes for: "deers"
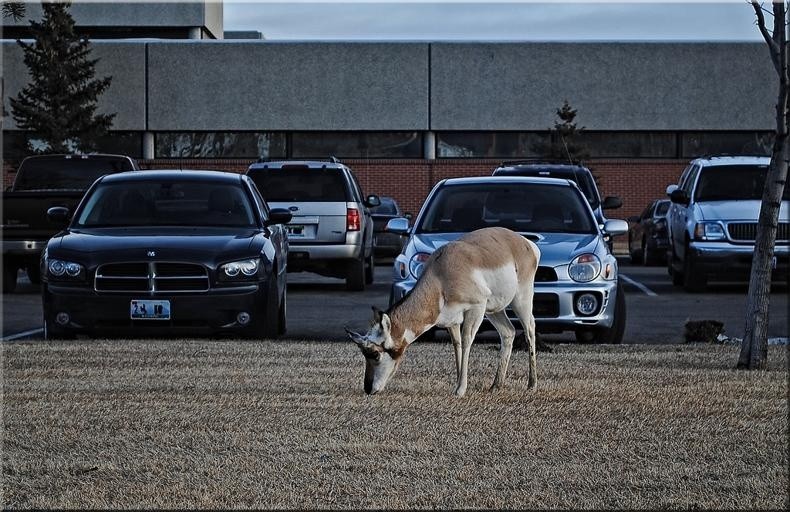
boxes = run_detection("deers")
[344,226,542,398]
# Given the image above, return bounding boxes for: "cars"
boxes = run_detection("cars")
[365,196,410,260]
[40,169,293,340]
[384,176,629,342]
[626,196,672,264]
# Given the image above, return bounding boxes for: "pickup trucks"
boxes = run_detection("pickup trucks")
[3,153,141,291]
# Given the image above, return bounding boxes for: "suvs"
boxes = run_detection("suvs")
[244,156,381,292]
[486,158,622,257]
[665,152,790,289]
